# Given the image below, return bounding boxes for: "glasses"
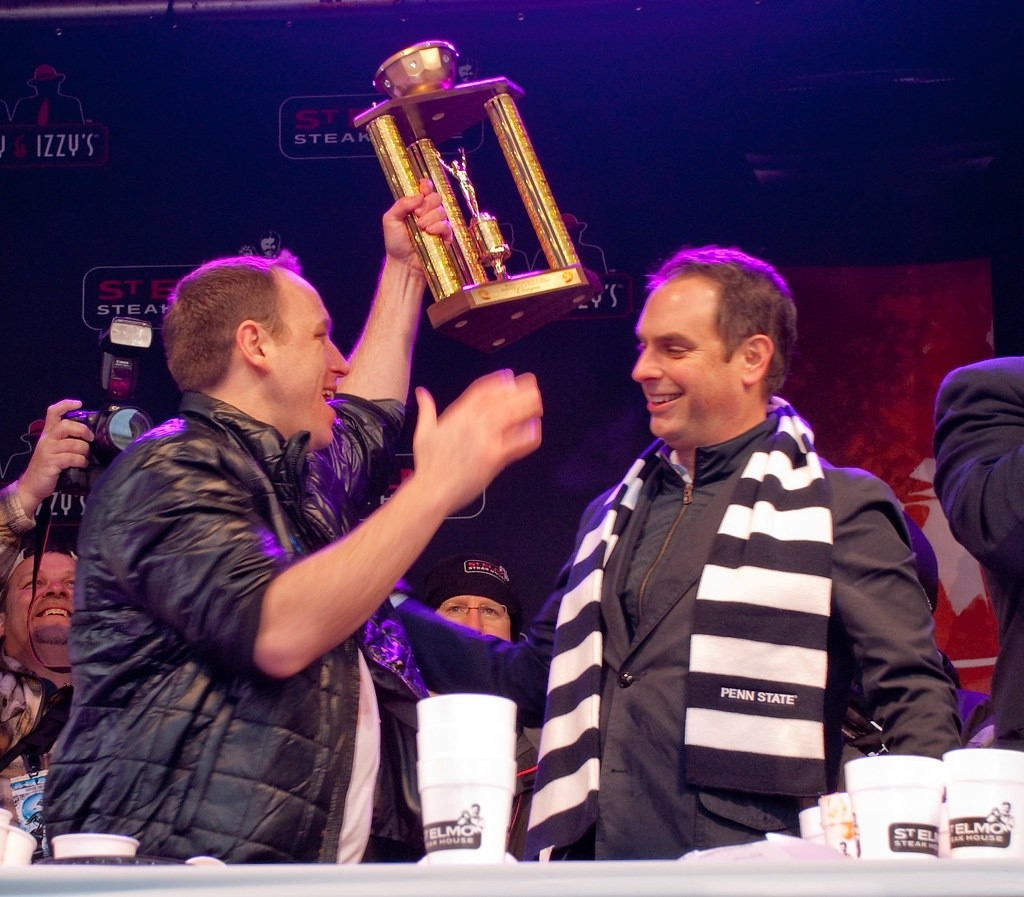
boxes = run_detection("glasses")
[442,603,507,621]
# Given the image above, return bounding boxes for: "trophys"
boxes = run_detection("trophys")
[353,38,601,339]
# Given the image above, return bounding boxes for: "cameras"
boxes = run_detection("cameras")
[61,315,155,490]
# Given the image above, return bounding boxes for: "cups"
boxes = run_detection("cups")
[0,807,140,865]
[414,692,519,869]
[798,749,1024,860]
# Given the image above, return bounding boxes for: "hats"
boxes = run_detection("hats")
[424,553,523,644]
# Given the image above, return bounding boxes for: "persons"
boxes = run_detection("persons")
[934,355,1024,755]
[419,554,516,642]
[44,176,546,866]
[0,397,97,822]
[366,247,959,861]
